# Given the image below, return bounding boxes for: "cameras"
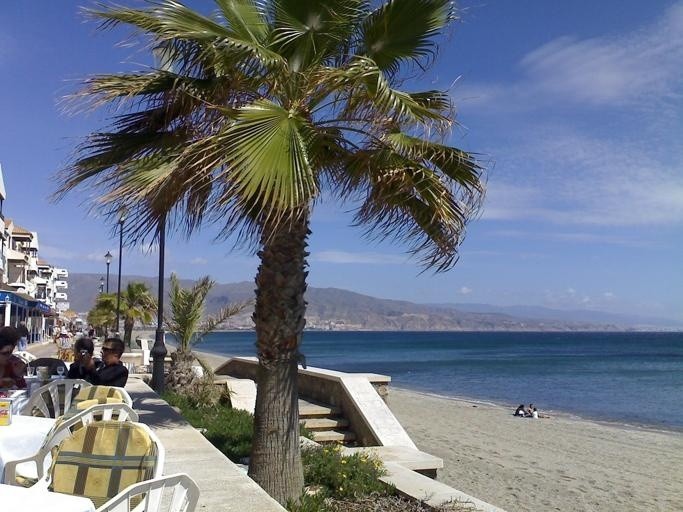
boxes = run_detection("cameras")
[80,349,88,360]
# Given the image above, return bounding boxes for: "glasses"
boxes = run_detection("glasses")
[101,346,114,353]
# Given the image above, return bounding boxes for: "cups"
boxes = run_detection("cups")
[26,366,35,377]
[35,365,48,378]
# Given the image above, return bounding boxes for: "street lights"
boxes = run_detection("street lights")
[105,250,112,341]
[114,202,131,334]
[97,276,105,338]
[149,39,181,392]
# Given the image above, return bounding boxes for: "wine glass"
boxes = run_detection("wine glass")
[56,366,64,379]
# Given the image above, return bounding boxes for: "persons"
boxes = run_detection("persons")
[0,325,26,389]
[515,404,528,417]
[15,320,29,351]
[79,337,128,389]
[527,403,534,414]
[525,407,538,419]
[66,336,100,379]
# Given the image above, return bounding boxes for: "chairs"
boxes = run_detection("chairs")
[0,352,199,511]
